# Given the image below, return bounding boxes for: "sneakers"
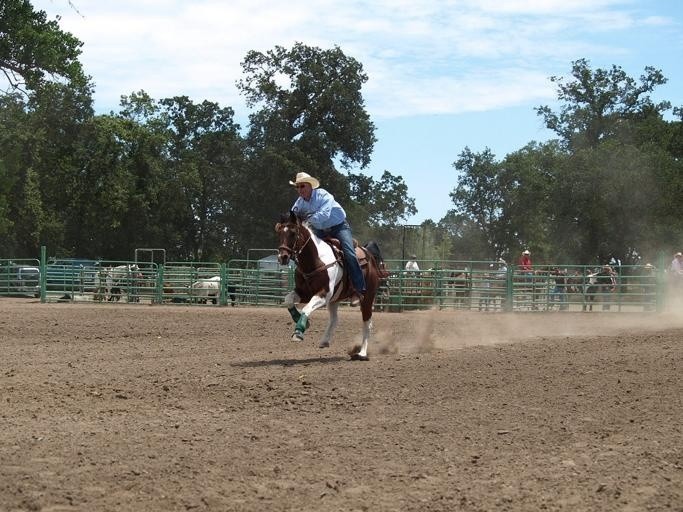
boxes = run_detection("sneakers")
[349,293,364,307]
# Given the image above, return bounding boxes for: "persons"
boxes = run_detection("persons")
[288,182,367,306]
[597,264,616,311]
[495,258,508,280]
[520,252,534,277]
[670,254,683,278]
[549,267,567,310]
[405,255,421,276]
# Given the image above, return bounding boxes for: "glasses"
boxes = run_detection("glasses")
[295,185,310,189]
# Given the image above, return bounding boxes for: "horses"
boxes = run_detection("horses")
[91,263,144,303]
[275,208,398,361]
[373,261,618,313]
[162,274,238,306]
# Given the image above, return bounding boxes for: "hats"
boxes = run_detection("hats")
[289,172,319,190]
[673,252,682,259]
[521,249,531,257]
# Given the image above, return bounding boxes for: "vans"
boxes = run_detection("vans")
[0,262,41,298]
